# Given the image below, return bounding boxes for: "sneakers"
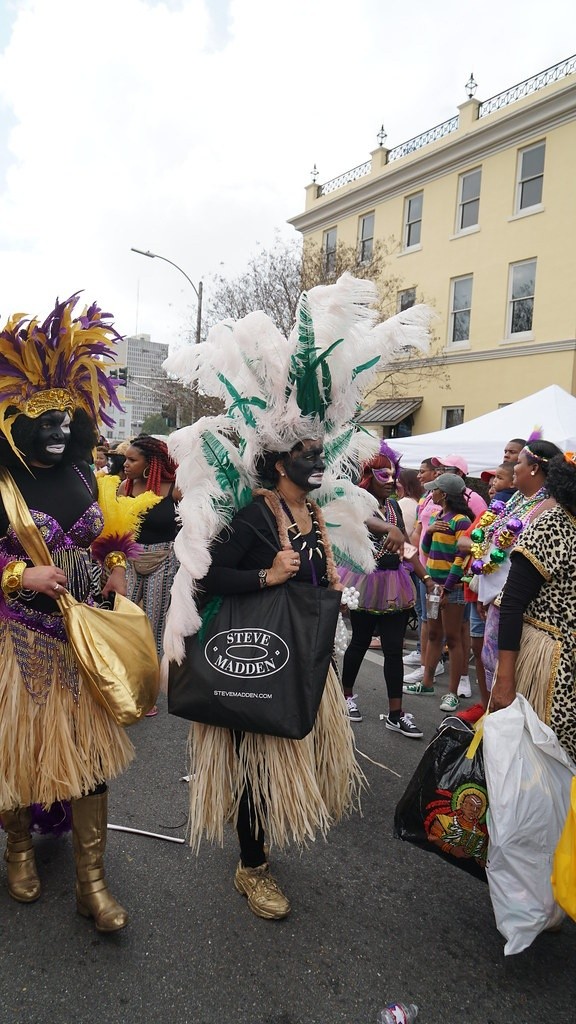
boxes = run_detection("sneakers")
[403,681,435,696]
[439,693,460,710]
[384,714,424,737]
[457,676,472,697]
[402,650,422,665]
[234,858,292,918]
[405,667,424,683]
[434,660,445,675]
[347,694,363,721]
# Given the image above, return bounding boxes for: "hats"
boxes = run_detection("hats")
[423,473,465,495]
[482,470,496,482]
[432,455,468,476]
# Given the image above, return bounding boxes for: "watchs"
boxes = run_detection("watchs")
[259,570,268,587]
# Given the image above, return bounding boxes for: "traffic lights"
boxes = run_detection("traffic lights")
[161,403,176,427]
[110,367,119,389]
[119,367,129,388]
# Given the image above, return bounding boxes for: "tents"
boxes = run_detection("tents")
[385,382,576,479]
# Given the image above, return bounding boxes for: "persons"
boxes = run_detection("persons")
[91,433,182,716]
[161,417,401,919]
[0,288,130,932]
[341,438,576,771]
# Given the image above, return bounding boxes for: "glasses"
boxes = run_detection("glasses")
[435,468,456,473]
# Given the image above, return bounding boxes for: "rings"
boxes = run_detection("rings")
[293,559,298,566]
[52,582,60,591]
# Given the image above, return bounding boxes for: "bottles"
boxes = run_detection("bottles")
[378,1002,419,1024]
[427,583,441,619]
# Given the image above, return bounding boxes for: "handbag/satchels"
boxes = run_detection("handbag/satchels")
[131,550,171,575]
[392,709,488,882]
[477,694,576,956]
[59,589,158,723]
[168,489,341,739]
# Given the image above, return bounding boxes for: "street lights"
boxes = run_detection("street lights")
[130,247,202,426]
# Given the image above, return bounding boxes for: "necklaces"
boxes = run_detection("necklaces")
[373,497,398,560]
[276,489,326,560]
[470,486,552,576]
[70,460,95,501]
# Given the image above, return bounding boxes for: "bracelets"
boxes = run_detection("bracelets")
[421,575,431,583]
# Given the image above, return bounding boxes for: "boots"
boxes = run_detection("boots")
[3,803,41,901]
[72,783,129,932]
